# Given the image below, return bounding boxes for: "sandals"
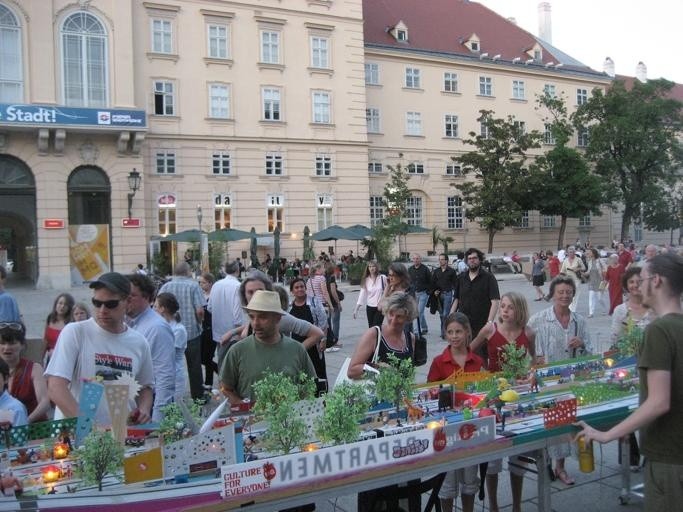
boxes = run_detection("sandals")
[554,468,575,485]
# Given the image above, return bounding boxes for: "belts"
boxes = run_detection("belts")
[439,289,452,293]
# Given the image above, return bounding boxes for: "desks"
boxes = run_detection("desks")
[0,348,644,512]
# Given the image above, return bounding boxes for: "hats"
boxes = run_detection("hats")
[89,272,131,296]
[239,289,288,316]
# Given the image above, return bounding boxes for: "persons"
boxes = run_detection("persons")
[407,252,431,337]
[287,278,329,397]
[448,249,501,361]
[318,250,330,261]
[470,293,535,512]
[238,271,326,352]
[427,313,486,511]
[0,267,21,324]
[640,240,682,260]
[427,253,462,341]
[195,272,219,391]
[136,263,146,275]
[220,291,318,404]
[236,257,246,278]
[125,271,177,428]
[611,266,657,348]
[559,244,587,314]
[149,292,191,403]
[40,293,74,369]
[0,321,51,440]
[579,249,608,319]
[1,357,29,446]
[347,291,419,378]
[305,262,334,337]
[155,261,210,406]
[279,257,310,282]
[326,263,344,351]
[378,263,411,320]
[69,303,90,323]
[353,259,386,329]
[218,283,289,347]
[524,276,593,485]
[44,273,156,429]
[576,237,609,257]
[612,235,639,266]
[601,254,627,316]
[529,238,561,302]
[206,258,251,379]
[264,252,272,272]
[572,251,682,511]
[339,248,361,283]
[502,242,521,273]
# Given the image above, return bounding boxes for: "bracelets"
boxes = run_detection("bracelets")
[320,336,327,342]
[229,328,238,336]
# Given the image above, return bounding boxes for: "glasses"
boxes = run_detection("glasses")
[0,322,26,338]
[638,275,657,286]
[91,297,124,309]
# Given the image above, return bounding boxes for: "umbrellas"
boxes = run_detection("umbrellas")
[160,228,260,243]
[400,224,432,253]
[267,227,281,282]
[249,227,261,268]
[301,225,378,258]
[362,245,375,277]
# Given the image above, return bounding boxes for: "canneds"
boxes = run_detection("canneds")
[579,435,595,474]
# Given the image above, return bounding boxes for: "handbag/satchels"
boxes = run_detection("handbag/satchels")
[329,275,345,304]
[405,286,428,367]
[542,270,547,282]
[332,326,383,402]
[312,296,338,348]
[576,259,589,284]
[310,277,331,319]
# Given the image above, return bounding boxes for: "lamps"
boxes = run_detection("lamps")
[126,169,141,199]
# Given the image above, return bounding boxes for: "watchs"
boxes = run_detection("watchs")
[486,320,494,325]
